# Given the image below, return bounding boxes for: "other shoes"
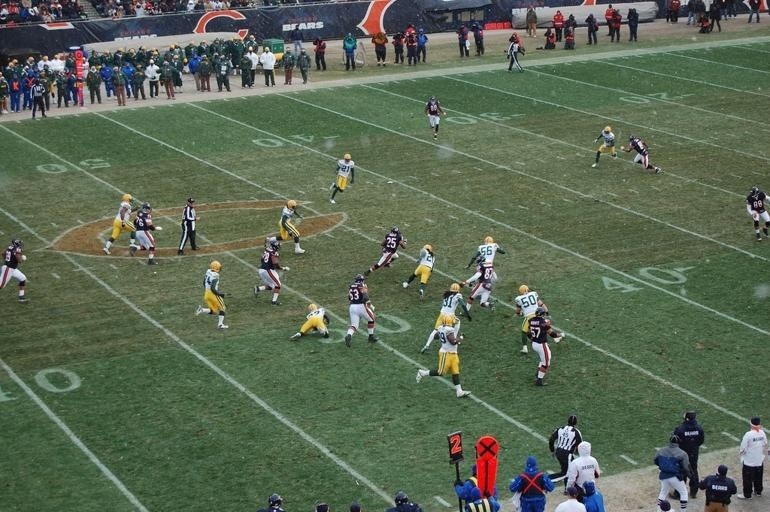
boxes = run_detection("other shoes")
[197,81,307,92]
[1,99,103,120]
[107,88,182,107]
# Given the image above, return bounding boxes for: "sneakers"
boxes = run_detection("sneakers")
[148,258,159,265]
[505,68,524,74]
[217,324,229,329]
[368,334,380,343]
[18,296,31,303]
[415,369,425,384]
[554,335,563,345]
[102,246,111,256]
[344,334,352,348]
[194,304,202,316]
[460,51,486,58]
[421,345,429,354]
[316,59,429,72]
[642,162,661,174]
[253,285,259,298]
[657,489,698,511]
[329,182,337,204]
[520,344,530,355]
[526,32,638,50]
[289,331,302,341]
[666,17,760,33]
[270,300,284,307]
[535,366,550,387]
[402,281,425,301]
[178,246,201,256]
[591,152,618,168]
[364,263,393,276]
[433,131,439,140]
[456,389,472,398]
[129,243,139,257]
[489,302,497,313]
[755,227,769,242]
[294,248,306,255]
[736,491,763,499]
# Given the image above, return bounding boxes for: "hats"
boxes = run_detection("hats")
[286,46,289,48]
[471,488,481,499]
[301,47,304,49]
[187,197,196,205]
[669,434,681,443]
[567,486,579,498]
[526,455,538,473]
[750,416,761,426]
[684,410,696,421]
[473,464,477,473]
[716,464,728,475]
[568,415,579,427]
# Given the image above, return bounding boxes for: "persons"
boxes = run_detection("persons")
[654,411,737,512]
[257,492,286,511]
[424,96,446,141]
[315,502,329,511]
[329,154,355,204]
[737,416,768,499]
[454,414,605,512]
[194,260,230,329]
[254,237,290,306]
[746,186,770,240]
[177,197,202,256]
[265,200,306,255]
[128,201,164,266]
[454,0,770,75]
[0,236,33,303]
[385,490,422,512]
[592,126,618,168]
[350,502,363,511]
[289,303,330,340]
[343,227,566,398]
[101,194,141,256]
[1,1,432,122]
[619,135,661,176]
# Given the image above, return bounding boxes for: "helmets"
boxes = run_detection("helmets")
[190,35,270,60]
[122,193,134,204]
[264,235,281,252]
[268,492,411,510]
[749,186,761,198]
[450,282,461,293]
[354,274,365,283]
[519,284,529,295]
[288,199,298,210]
[210,260,223,272]
[344,154,353,163]
[423,244,433,253]
[604,124,638,141]
[535,307,547,317]
[142,201,153,215]
[430,96,437,104]
[11,238,23,249]
[442,314,454,327]
[391,225,401,234]
[308,304,318,312]
[476,236,495,264]
[7,53,70,68]
[91,43,188,65]
